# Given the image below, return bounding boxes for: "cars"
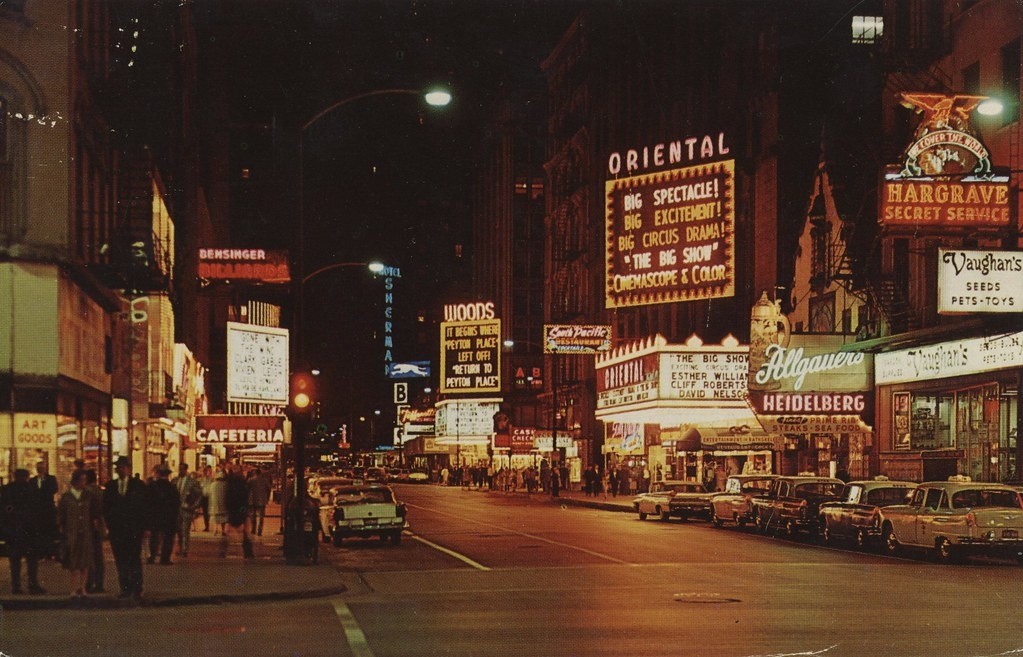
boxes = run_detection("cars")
[632,480,713,524]
[708,472,783,532]
[749,469,847,540]
[871,474,1023,566]
[817,473,920,553]
[304,457,430,549]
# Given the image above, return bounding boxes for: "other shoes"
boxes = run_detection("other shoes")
[119,587,141,597]
[147,557,154,563]
[12,588,45,595]
[160,560,173,565]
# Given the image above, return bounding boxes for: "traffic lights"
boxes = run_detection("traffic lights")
[291,372,313,414]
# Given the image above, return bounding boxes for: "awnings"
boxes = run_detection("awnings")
[676,426,781,452]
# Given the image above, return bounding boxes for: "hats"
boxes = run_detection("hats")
[114,455,132,467]
[152,463,173,473]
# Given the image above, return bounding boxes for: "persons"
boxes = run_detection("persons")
[279,473,330,542]
[435,464,571,498]
[0,448,272,601]
[583,461,665,498]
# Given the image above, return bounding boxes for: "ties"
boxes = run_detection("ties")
[179,478,182,491]
[120,479,124,494]
